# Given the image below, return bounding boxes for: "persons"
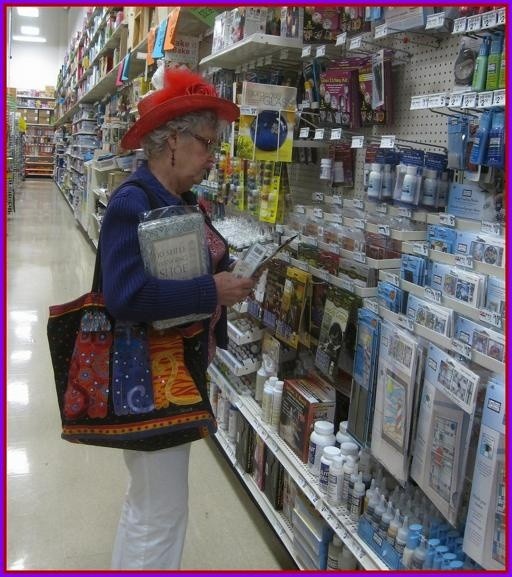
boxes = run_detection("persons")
[90,68,265,571]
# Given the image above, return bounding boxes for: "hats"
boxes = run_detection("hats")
[121,77,240,149]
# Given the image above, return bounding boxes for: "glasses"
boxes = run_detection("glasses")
[182,128,218,153]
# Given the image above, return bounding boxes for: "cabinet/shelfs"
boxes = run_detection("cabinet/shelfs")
[7,95,55,215]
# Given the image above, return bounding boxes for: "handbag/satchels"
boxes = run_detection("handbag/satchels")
[46,292,218,452]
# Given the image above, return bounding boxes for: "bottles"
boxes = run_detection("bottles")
[25,126,54,153]
[333,162,344,183]
[307,420,485,572]
[319,158,331,181]
[470,41,506,169]
[254,366,285,430]
[363,163,448,209]
